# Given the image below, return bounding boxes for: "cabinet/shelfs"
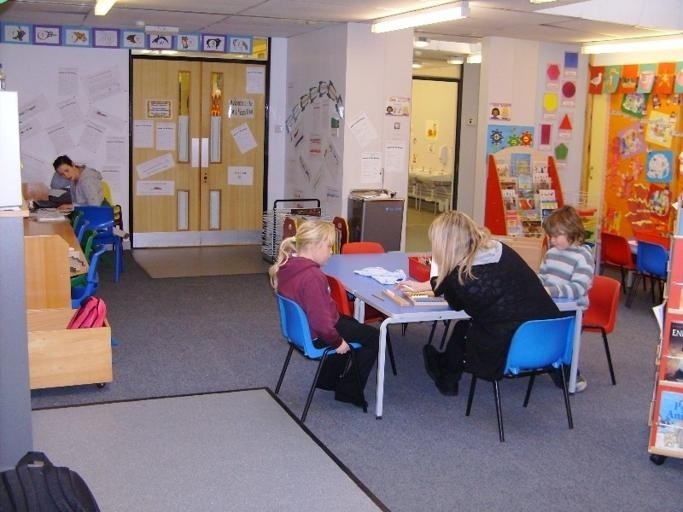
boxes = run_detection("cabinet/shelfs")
[345,194,406,252]
[562,189,599,275]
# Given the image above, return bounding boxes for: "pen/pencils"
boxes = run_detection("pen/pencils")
[395,283,414,292]
[372,293,384,301]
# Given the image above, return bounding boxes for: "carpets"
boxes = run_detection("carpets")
[28,381,395,512]
[129,238,273,281]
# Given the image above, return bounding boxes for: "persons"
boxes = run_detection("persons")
[52,155,105,211]
[393,211,561,395]
[268,218,379,409]
[536,205,594,395]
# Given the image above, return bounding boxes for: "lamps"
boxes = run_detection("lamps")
[91,0,118,17]
[444,56,466,65]
[413,35,429,48]
[578,32,682,56]
[368,0,471,35]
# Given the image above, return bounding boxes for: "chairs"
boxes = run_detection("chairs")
[341,240,383,257]
[599,230,650,302]
[323,271,401,378]
[65,178,132,308]
[625,240,670,311]
[575,272,622,389]
[462,314,582,445]
[271,290,373,426]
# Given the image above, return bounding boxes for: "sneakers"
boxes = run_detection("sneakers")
[564,365,589,393]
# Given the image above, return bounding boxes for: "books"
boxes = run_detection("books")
[494,151,559,241]
[403,290,449,306]
[662,322,683,382]
[34,208,64,221]
[655,391,683,452]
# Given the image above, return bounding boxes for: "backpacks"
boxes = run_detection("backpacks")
[0,450,99,511]
[65,294,105,329]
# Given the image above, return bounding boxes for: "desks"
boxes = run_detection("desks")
[321,252,584,419]
[23,190,90,315]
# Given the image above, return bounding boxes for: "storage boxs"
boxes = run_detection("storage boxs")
[26,306,116,393]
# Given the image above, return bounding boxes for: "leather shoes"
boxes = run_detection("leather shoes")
[317,373,371,408]
[424,345,460,396]
